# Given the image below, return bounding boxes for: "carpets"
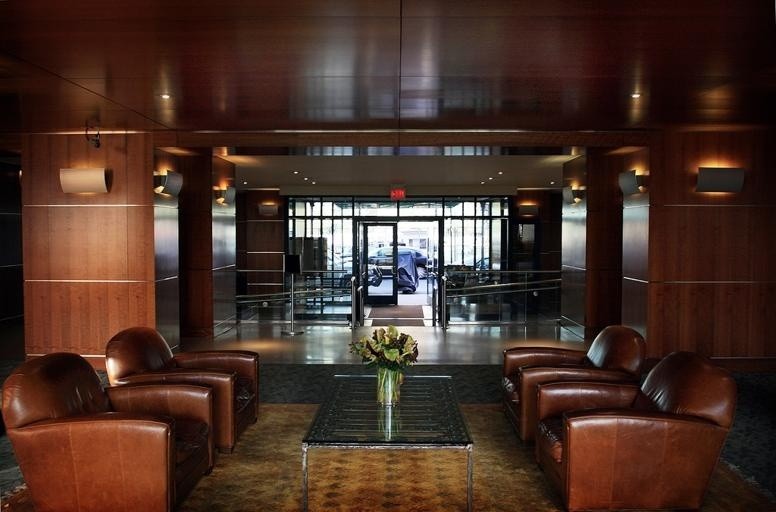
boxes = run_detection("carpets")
[367,305,425,326]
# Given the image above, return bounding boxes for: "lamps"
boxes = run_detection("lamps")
[617,170,644,196]
[59,167,109,195]
[561,185,581,206]
[518,205,539,216]
[256,202,279,217]
[154,169,184,198]
[696,166,745,194]
[216,186,238,206]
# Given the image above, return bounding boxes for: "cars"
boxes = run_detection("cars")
[305,242,437,294]
[476,257,489,270]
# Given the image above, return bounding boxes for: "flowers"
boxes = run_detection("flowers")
[346,323,419,401]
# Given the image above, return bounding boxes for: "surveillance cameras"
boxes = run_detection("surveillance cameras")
[91,138,101,148]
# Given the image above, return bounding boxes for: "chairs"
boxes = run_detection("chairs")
[501,324,647,449]
[535,351,740,511]
[2,352,214,510]
[105,326,261,454]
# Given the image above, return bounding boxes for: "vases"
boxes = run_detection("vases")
[376,364,404,411]
